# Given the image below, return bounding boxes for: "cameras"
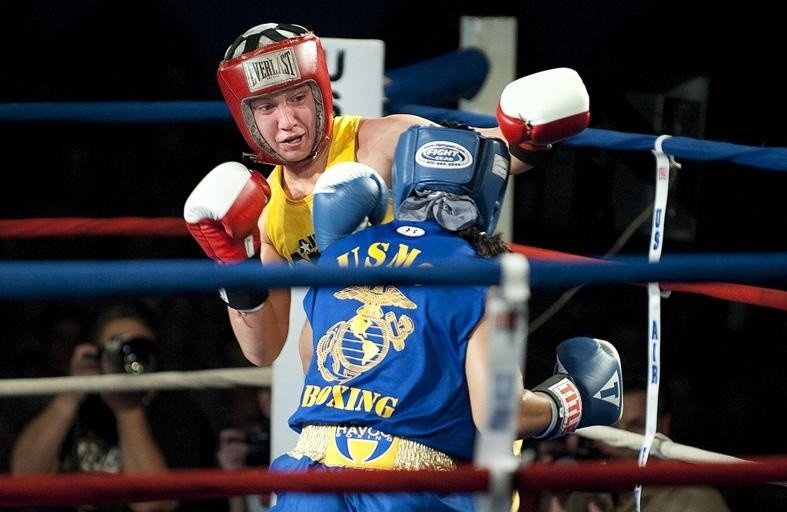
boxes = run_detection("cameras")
[96,331,157,398]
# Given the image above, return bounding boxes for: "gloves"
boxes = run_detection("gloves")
[497,68,589,168]
[183,162,273,312]
[313,163,388,254]
[531,336,624,441]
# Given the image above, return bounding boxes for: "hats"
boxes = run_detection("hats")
[392,124,510,236]
[216,22,335,169]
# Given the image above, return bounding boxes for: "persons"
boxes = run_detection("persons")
[512,368,732,512]
[183,23,593,376]
[0,298,271,512]
[267,124,623,512]
